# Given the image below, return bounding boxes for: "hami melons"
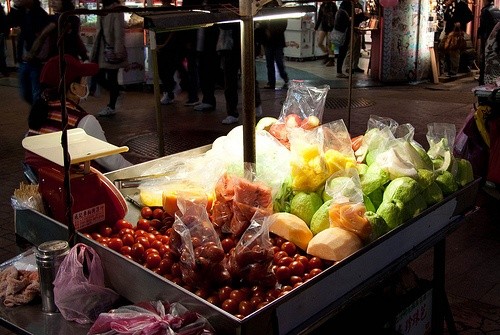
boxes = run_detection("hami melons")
[266,212,364,260]
[139,185,214,219]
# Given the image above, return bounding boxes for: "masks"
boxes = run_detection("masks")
[74,84,90,106]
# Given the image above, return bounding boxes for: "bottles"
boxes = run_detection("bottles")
[35,240,71,315]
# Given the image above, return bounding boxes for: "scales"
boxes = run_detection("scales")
[21,128,129,235]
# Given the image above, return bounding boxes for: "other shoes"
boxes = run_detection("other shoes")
[323,54,336,67]
[345,65,364,74]
[184,99,200,106]
[160,96,175,105]
[336,72,349,78]
[193,103,216,112]
[98,104,117,116]
[264,84,275,89]
[282,82,289,89]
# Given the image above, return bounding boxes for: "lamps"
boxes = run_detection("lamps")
[213,5,318,25]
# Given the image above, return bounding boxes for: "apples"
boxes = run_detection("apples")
[269,114,318,148]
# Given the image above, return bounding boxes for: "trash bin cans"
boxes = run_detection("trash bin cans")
[474,87,500,192]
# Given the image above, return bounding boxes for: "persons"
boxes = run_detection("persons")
[4,0,49,99]
[26,0,88,63]
[182,0,217,111]
[346,0,364,74]
[25,56,134,177]
[261,0,289,90]
[0,4,9,78]
[218,0,262,123]
[94,0,128,115]
[313,1,337,66]
[442,0,474,74]
[478,0,500,85]
[153,0,180,104]
[334,0,349,78]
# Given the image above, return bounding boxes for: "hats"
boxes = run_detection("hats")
[39,54,100,84]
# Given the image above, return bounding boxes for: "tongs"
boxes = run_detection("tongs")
[113,170,178,189]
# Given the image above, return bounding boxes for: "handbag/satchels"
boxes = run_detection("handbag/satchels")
[103,46,128,65]
[445,24,467,49]
[52,243,119,324]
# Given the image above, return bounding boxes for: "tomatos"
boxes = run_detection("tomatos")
[81,207,338,320]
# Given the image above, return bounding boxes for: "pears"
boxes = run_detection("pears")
[273,127,474,240]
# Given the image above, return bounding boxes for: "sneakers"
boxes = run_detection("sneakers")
[255,105,263,118]
[222,116,239,125]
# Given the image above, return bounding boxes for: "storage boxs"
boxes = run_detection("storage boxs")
[11,143,486,334]
[385,288,435,335]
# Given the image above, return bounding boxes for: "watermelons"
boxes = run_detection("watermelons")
[212,172,273,236]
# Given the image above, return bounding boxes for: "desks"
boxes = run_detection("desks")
[0,205,483,335]
[76,30,148,97]
